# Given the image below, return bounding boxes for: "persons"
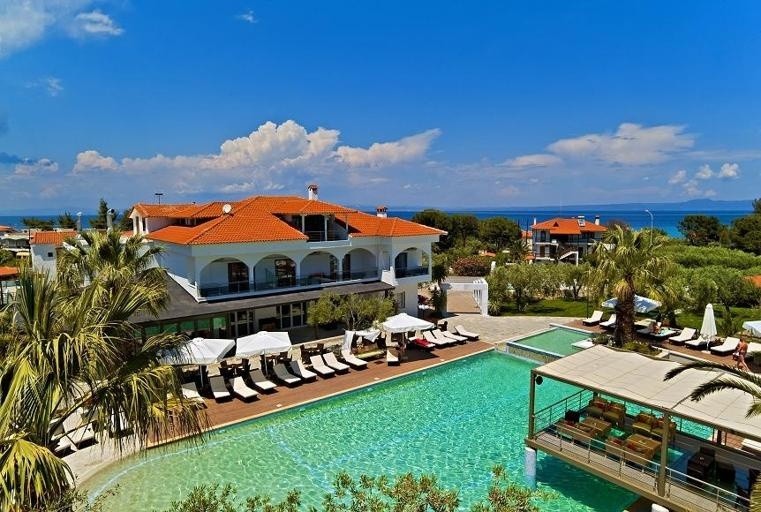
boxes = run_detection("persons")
[736,338,751,372]
[438,321,449,331]
[649,321,661,335]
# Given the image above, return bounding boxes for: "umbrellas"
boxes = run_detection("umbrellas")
[742,318,761,340]
[233,330,294,380]
[699,303,719,350]
[159,336,236,390]
[600,293,663,316]
[376,312,437,353]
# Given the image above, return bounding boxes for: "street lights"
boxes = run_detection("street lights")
[645,209,654,245]
[155,192,163,204]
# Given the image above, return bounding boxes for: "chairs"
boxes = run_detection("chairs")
[556,396,677,469]
[582,309,761,363]
[47,404,134,456]
[175,343,369,408]
[408,318,481,356]
